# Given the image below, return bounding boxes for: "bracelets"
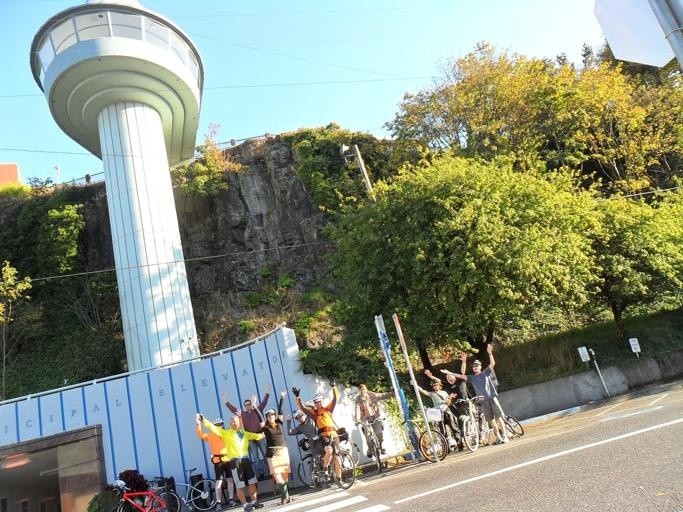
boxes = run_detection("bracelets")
[488,353,492,355]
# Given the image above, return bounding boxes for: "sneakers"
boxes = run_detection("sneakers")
[493,434,508,444]
[368,449,372,458]
[244,502,264,512]
[216,503,222,512]
[229,499,238,507]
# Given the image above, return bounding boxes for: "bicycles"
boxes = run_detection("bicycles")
[110,485,180,511]
[401,395,524,463]
[297,431,360,490]
[152,468,219,512]
[356,418,387,473]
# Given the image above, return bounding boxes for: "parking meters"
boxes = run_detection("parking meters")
[589,348,610,398]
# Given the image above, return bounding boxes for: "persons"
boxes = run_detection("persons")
[424,352,483,437]
[410,379,464,454]
[354,383,395,458]
[220,383,270,478]
[440,344,510,445]
[252,391,293,506]
[291,376,343,488]
[195,414,238,511]
[286,403,329,488]
[198,411,266,512]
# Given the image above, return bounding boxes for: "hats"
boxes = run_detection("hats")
[472,360,481,367]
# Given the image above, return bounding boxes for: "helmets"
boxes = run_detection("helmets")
[214,417,224,425]
[312,393,323,401]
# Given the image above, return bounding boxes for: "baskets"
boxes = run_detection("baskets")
[190,474,203,492]
[426,408,442,423]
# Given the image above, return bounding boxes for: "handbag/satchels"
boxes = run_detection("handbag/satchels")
[337,428,349,441]
